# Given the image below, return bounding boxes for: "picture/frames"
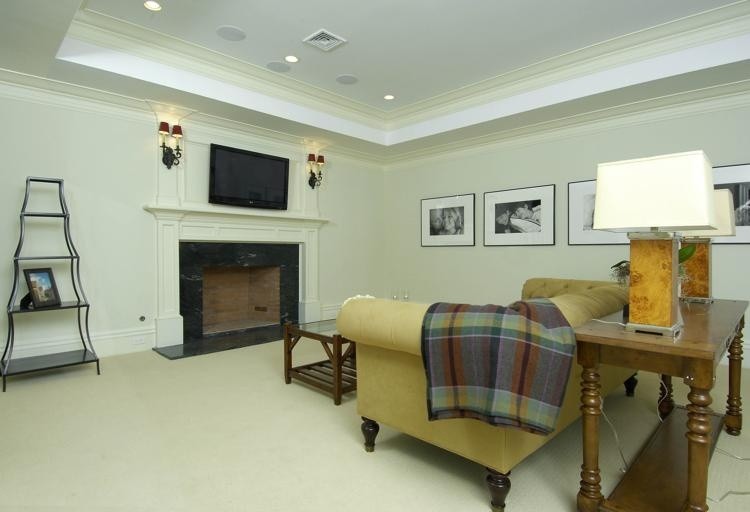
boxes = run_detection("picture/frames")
[711,163,750,244]
[568,178,630,245]
[483,184,555,246]
[20,267,62,309]
[421,193,475,247]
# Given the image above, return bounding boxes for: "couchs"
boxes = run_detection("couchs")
[336,279,639,512]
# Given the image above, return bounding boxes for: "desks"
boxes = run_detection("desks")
[573,298,750,512]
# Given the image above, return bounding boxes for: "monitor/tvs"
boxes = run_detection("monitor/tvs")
[208,143,291,211]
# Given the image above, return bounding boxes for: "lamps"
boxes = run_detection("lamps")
[592,150,718,338]
[308,153,324,189]
[159,121,183,169]
[675,188,735,304]
